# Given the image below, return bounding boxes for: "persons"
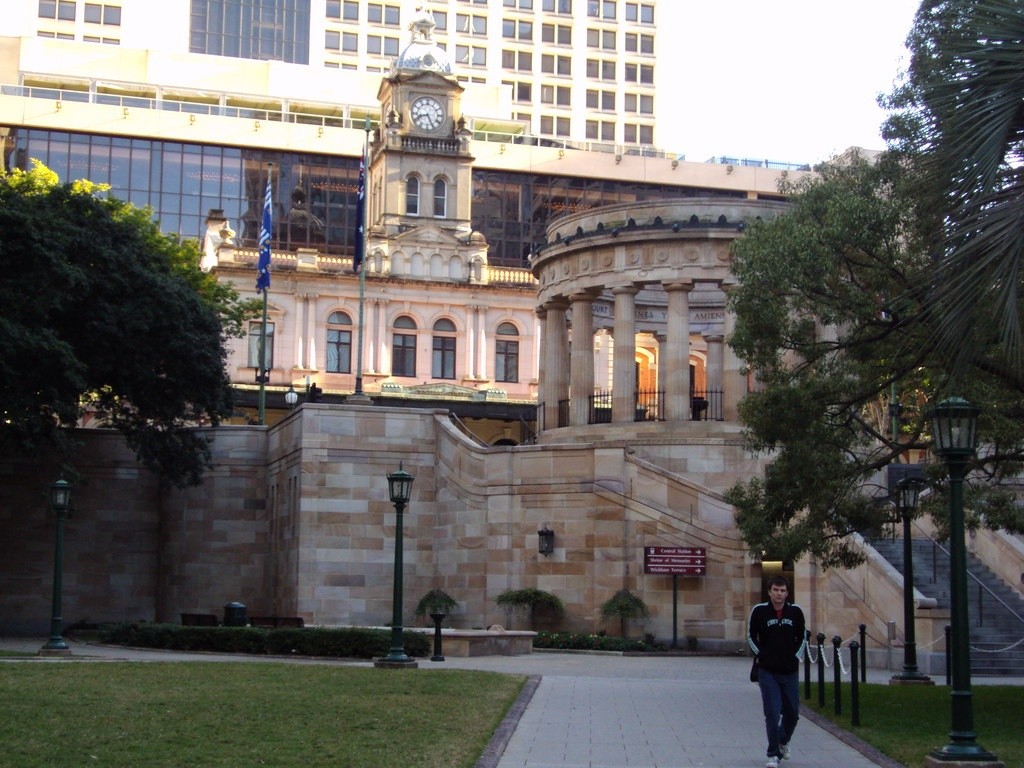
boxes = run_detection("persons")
[745,573,807,768]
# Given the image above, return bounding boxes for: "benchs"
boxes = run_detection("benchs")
[250,616,303,629]
[182,613,218,627]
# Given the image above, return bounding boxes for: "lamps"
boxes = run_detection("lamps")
[727,166,733,174]
[558,150,564,159]
[500,145,505,154]
[538,525,554,557]
[672,223,680,233]
[563,238,570,247]
[55,102,61,113]
[615,156,622,165]
[672,161,678,170]
[318,128,323,138]
[254,121,259,132]
[611,228,618,237]
[190,115,195,126]
[737,222,744,234]
[782,171,787,179]
[122,108,128,119]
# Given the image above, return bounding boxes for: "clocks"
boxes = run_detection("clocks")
[385,104,391,122]
[409,96,445,133]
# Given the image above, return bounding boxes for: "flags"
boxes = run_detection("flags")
[353,154,365,272]
[255,179,273,294]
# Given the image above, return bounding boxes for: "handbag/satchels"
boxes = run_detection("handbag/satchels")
[750,663,760,682]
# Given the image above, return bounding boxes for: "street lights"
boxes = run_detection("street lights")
[373,460,420,670]
[888,473,937,686]
[927,397,1006,768]
[37,473,74,657]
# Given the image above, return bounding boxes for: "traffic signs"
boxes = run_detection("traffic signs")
[642,543,706,576]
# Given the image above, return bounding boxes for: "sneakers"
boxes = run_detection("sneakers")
[779,743,791,759]
[766,756,779,768]
